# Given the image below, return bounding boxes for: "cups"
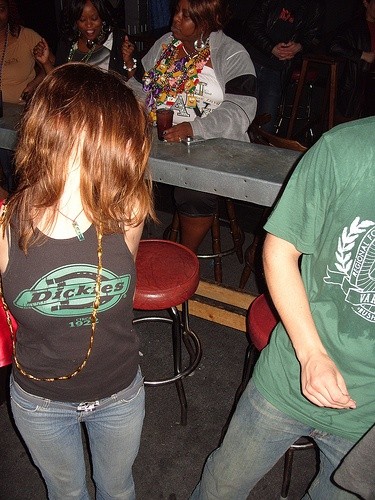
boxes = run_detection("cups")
[155,108,174,140]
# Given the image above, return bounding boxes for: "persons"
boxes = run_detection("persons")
[246,0,375,145]
[189,114,375,500]
[0,0,55,193]
[33,0,145,78]
[0,63,155,500]
[126,0,259,254]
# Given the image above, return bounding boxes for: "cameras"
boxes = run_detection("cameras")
[180,135,204,146]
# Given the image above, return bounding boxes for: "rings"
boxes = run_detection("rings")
[179,137,182,143]
[127,44,131,48]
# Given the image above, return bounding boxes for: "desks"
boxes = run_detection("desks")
[286,47,346,137]
[0,102,304,333]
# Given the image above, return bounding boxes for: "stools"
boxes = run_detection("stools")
[133,66,321,500]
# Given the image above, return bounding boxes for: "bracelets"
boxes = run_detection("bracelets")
[123,58,137,71]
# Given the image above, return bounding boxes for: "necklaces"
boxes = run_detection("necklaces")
[57,209,85,241]
[143,40,211,126]
[2,207,103,382]
[0,28,7,87]
[68,41,96,63]
